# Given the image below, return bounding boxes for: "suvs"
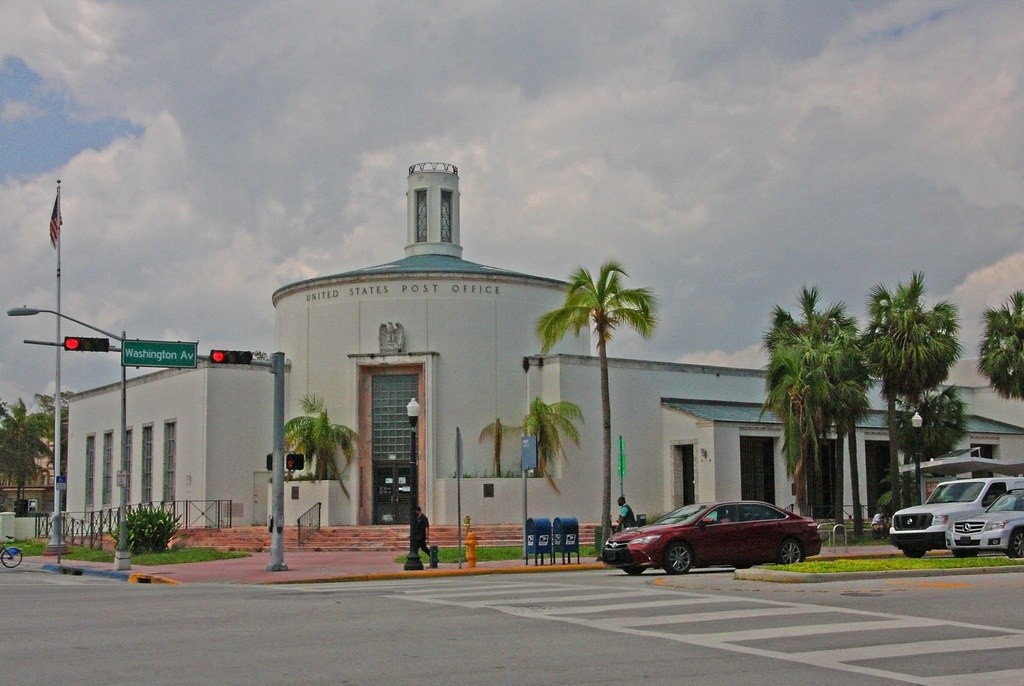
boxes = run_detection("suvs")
[889,475,1024,558]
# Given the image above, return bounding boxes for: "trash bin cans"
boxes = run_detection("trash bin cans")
[595,526,622,560]
[553,517,580,565]
[526,518,552,566]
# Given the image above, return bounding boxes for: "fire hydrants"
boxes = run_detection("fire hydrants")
[463,531,479,567]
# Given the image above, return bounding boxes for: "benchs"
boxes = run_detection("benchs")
[818,529,874,543]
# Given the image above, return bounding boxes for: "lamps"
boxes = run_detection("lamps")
[701,448,707,459]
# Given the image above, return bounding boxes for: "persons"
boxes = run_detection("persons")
[408,506,430,558]
[872,512,888,540]
[616,496,636,531]
[718,509,726,521]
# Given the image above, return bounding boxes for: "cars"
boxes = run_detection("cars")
[945,488,1024,558]
[602,500,822,575]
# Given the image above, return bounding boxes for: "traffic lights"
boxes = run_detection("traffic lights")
[286,454,305,470]
[210,350,253,365]
[64,336,110,352]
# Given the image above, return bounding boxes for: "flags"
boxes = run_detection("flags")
[49,192,63,251]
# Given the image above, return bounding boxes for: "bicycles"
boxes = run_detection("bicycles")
[0,536,22,568]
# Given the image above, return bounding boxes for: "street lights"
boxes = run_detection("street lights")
[404,397,424,572]
[911,412,922,505]
[8,304,132,570]
